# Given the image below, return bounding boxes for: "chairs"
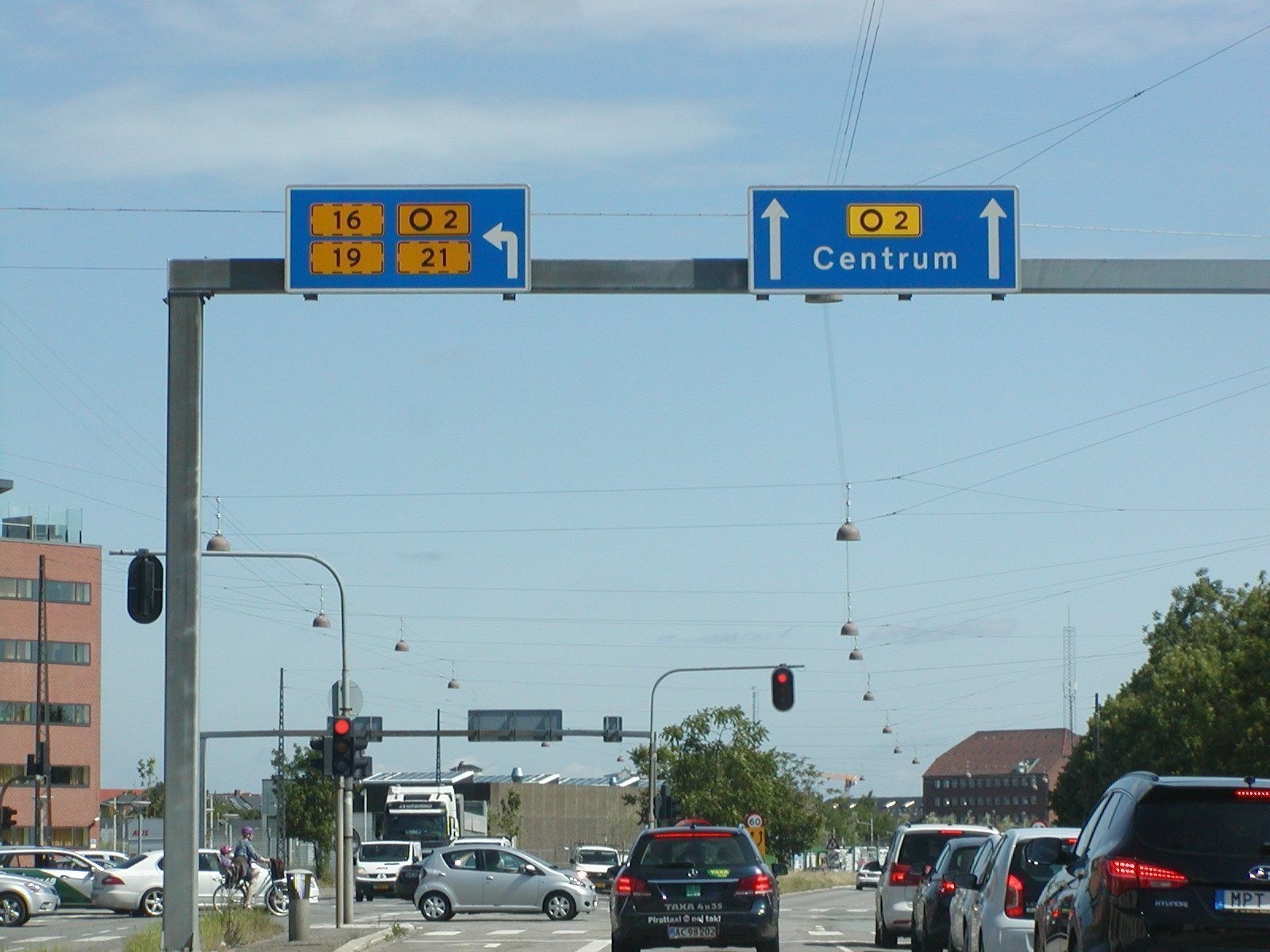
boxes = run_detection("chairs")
[1024,860,1049,877]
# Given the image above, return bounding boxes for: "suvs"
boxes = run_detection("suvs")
[1024,771,1270,952]
[354,836,626,923]
[864,820,1000,950]
[607,823,789,952]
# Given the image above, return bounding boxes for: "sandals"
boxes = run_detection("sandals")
[243,903,254,911]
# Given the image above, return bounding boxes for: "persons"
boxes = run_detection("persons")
[487,851,525,873]
[217,845,245,888]
[233,826,271,911]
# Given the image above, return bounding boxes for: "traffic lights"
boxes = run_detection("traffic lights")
[771,667,795,713]
[332,716,351,775]
[667,796,681,818]
[350,736,368,776]
[2,806,17,829]
[309,736,332,776]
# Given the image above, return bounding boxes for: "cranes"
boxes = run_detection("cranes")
[798,770,867,799]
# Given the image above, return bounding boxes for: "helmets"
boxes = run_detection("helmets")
[220,846,232,854]
[241,826,253,834]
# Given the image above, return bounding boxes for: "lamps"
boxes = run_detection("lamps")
[849,636,864,661]
[841,594,860,637]
[312,584,330,629]
[859,775,866,781]
[541,741,550,747]
[863,673,875,701]
[448,660,459,688]
[963,762,973,778]
[882,711,894,734]
[912,746,921,765]
[206,495,231,552]
[836,481,863,541]
[393,617,410,652]
[806,294,845,305]
[893,732,903,754]
[617,742,625,762]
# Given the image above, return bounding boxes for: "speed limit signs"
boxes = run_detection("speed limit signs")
[745,811,764,828]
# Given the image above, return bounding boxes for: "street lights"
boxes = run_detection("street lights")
[223,813,239,847]
[31,796,53,846]
[130,801,152,853]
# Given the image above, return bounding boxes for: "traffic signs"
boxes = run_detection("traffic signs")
[747,183,1021,295]
[283,181,533,295]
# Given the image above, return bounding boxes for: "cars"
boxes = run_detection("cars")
[90,849,319,918]
[909,837,988,952]
[0,844,132,927]
[855,864,882,890]
[941,834,1007,952]
[954,826,1083,952]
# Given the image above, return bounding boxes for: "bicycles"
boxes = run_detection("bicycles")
[212,861,290,917]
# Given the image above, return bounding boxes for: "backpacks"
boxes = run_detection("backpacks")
[268,857,284,879]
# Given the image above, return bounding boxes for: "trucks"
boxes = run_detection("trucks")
[374,784,489,859]
